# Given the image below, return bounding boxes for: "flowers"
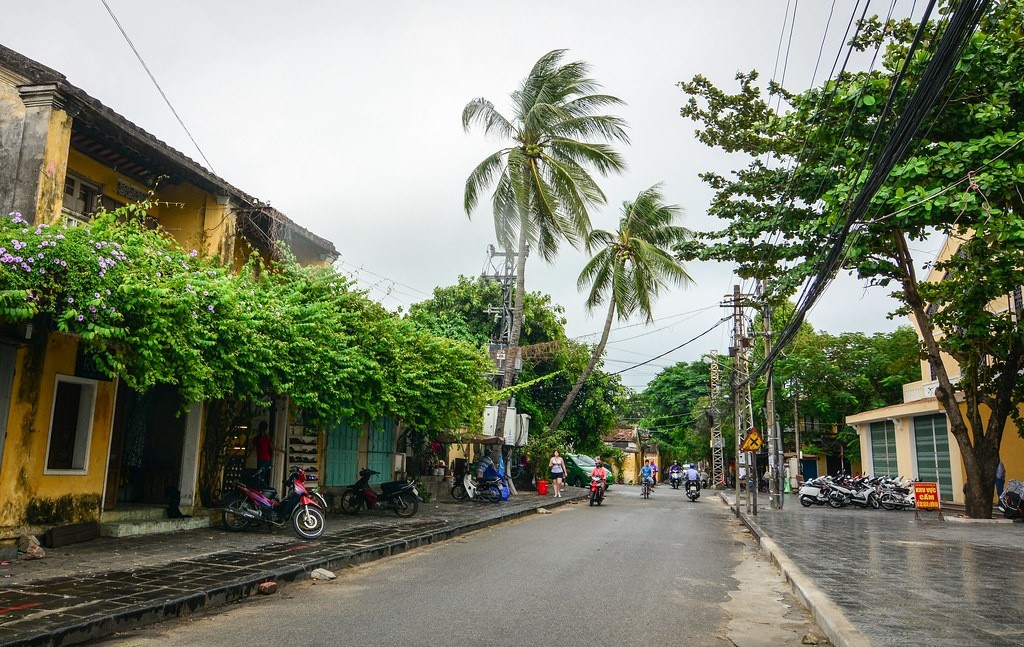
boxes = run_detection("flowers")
[432,442,442,468]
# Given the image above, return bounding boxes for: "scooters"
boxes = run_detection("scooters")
[639,471,659,499]
[586,473,606,507]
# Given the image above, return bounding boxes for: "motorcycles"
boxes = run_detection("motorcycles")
[668,469,683,489]
[798,469,934,512]
[683,473,701,502]
[699,476,710,489]
[218,465,326,540]
[451,458,504,504]
[340,467,423,519]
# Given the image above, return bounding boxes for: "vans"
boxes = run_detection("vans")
[560,452,615,491]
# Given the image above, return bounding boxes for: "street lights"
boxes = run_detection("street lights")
[703,356,758,515]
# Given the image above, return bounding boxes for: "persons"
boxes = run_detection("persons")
[476,448,497,484]
[993,463,1006,509]
[521,451,531,468]
[669,459,683,485]
[548,450,568,498]
[588,460,607,505]
[244,420,285,490]
[684,464,701,495]
[638,459,658,495]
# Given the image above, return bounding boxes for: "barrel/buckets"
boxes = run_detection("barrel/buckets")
[433,468,444,475]
[537,481,549,495]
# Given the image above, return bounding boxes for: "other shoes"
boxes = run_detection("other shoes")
[553,493,562,497]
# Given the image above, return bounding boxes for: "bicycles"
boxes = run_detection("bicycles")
[251,465,328,531]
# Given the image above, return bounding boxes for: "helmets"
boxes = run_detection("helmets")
[690,464,695,469]
[595,459,602,468]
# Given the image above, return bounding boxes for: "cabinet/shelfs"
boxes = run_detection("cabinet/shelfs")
[286,424,321,499]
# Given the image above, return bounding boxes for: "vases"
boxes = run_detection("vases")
[438,468,444,476]
[432,469,437,476]
